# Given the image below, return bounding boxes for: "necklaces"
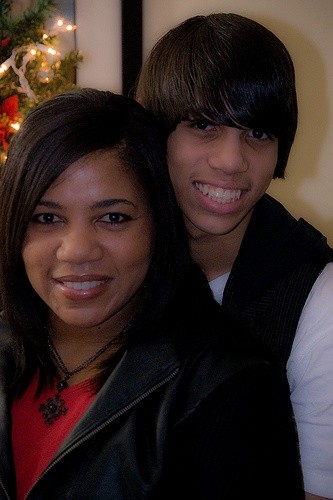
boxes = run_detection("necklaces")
[38,325,130,426]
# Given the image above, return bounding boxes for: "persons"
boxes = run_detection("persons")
[134,13,333,500]
[0,87,305,499]
